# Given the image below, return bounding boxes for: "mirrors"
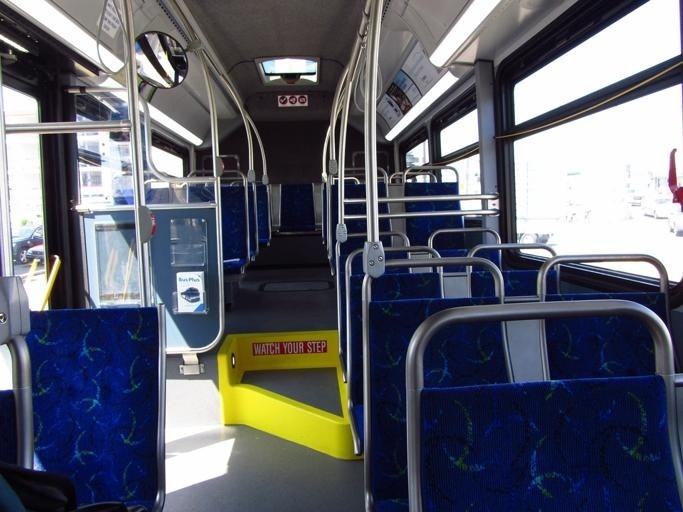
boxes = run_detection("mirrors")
[133,30,188,91]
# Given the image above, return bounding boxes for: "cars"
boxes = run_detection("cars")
[12,225,44,265]
[631,194,683,236]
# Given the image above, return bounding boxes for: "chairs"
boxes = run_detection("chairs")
[321,166,681,512]
[276,182,317,235]
[110,175,270,295]
[2,304,173,511]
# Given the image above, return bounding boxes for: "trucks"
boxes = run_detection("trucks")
[451,154,560,244]
